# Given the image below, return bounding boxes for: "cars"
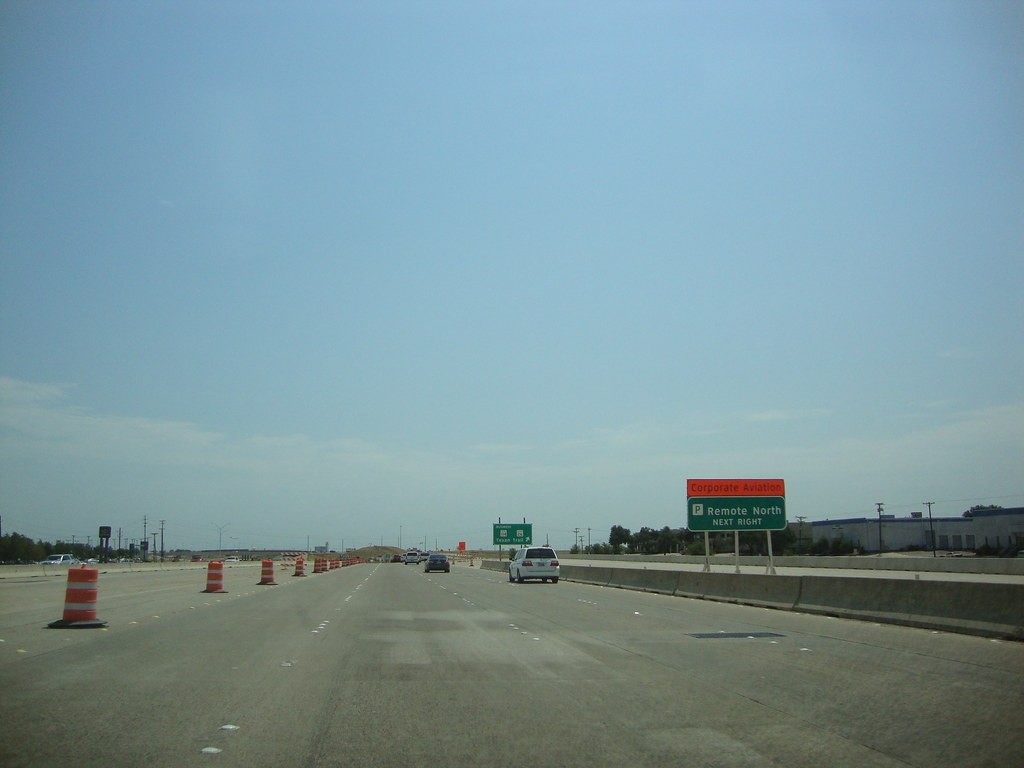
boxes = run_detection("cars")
[226,556,239,563]
[84,558,99,563]
[390,554,402,563]
[400,553,407,562]
[113,557,131,562]
[419,552,430,563]
[191,555,203,563]
[424,554,451,573]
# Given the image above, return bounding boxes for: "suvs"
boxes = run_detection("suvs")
[41,553,76,566]
[507,545,561,584]
[405,552,420,565]
